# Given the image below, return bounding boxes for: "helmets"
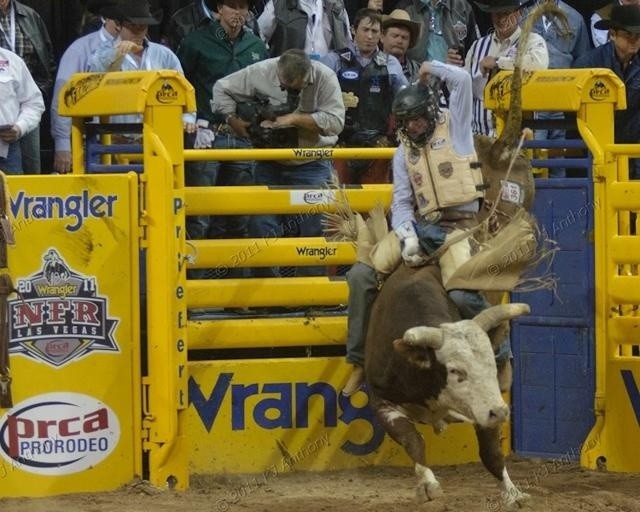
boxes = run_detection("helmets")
[391,84,433,118]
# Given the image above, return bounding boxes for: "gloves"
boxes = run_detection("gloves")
[394,234,422,265]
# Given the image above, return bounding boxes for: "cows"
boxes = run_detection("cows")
[364,2,572,510]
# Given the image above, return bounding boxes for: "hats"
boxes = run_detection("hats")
[122,0,159,27]
[476,0,532,14]
[378,9,419,46]
[593,5,640,35]
[87,2,122,18]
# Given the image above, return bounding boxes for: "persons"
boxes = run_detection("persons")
[257,0,353,77]
[157,1,259,51]
[518,0,591,178]
[211,49,347,312]
[334,8,412,184]
[569,5,640,181]
[176,0,273,316]
[590,0,640,49]
[322,60,539,397]
[0,0,59,174]
[83,0,197,151]
[0,47,46,175]
[464,0,548,140]
[401,0,482,66]
[49,0,125,174]
[377,9,423,85]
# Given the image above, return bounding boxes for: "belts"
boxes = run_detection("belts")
[203,118,237,135]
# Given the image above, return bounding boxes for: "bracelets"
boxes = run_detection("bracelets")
[225,113,236,125]
[495,56,500,71]
